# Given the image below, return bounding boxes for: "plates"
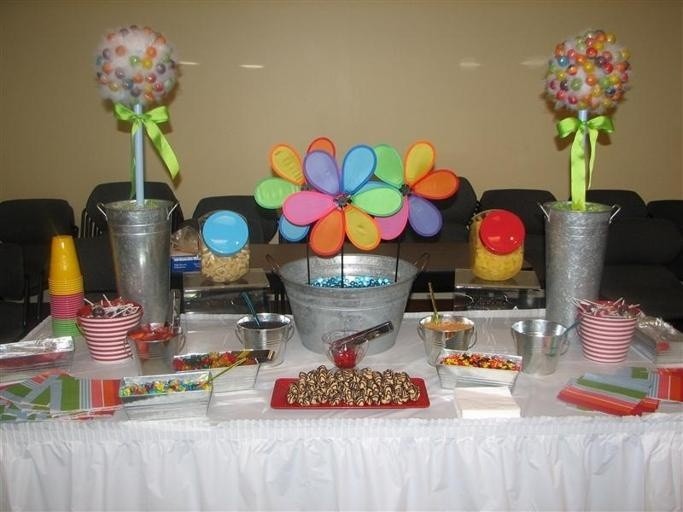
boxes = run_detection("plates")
[269,375,429,411]
[117,371,214,421]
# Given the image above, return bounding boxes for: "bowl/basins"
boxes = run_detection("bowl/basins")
[173,347,260,392]
[632,319,682,365]
[434,348,522,393]
[0,336,74,370]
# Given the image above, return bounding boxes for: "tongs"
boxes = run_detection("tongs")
[332,321,393,349]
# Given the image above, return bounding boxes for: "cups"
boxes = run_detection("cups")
[48,234,85,341]
[574,300,640,364]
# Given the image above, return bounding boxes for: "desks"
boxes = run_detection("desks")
[0,309,683,512]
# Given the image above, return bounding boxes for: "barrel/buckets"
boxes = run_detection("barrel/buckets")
[578,310,638,363]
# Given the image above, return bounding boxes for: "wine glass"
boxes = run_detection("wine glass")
[321,329,369,377]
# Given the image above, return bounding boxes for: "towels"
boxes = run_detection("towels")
[454,386,520,419]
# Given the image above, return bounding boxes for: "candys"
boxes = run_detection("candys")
[286,365,420,405]
[571,296,640,319]
[92,24,182,109]
[82,293,140,319]
[174,351,260,370]
[441,352,520,371]
[119,378,208,396]
[543,27,631,115]
